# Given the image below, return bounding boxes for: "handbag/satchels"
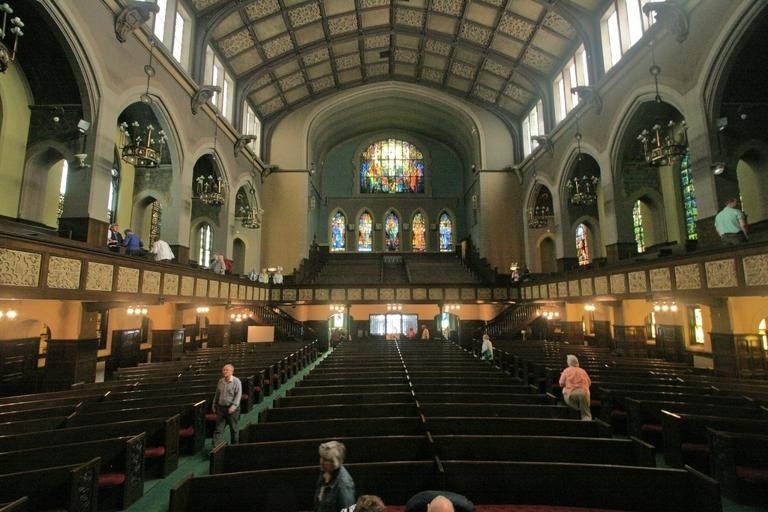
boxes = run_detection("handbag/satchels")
[483,351,491,359]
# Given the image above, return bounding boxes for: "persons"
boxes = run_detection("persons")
[328,327,349,350]
[151,236,175,263]
[312,440,355,512]
[420,325,428,339]
[122,228,143,256]
[340,495,388,512]
[406,327,415,336]
[404,490,474,512]
[206,363,242,456]
[480,334,493,365]
[558,354,591,421]
[209,253,283,285]
[713,196,749,245]
[107,223,123,252]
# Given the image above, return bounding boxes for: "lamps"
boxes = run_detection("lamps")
[0,307,17,320]
[527,139,549,229]
[196,93,225,207]
[0,3,24,74]
[127,305,148,315]
[240,139,264,229]
[536,303,561,320]
[637,11,688,164]
[566,93,599,206]
[711,162,727,176]
[119,10,168,167]
[653,300,678,312]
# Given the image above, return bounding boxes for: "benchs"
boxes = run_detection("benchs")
[0,336,768,512]
